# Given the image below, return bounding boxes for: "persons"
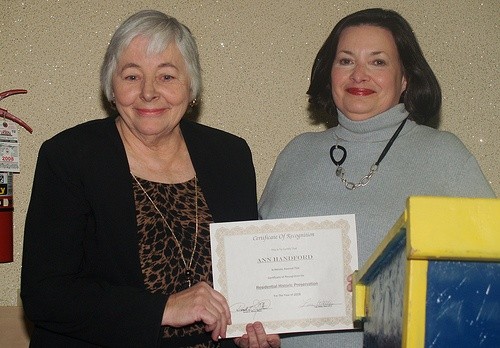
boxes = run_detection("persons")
[20,11,281,348]
[257,7,496,348]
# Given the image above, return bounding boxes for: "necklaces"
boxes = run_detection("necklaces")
[330,119,408,190]
[130,172,199,288]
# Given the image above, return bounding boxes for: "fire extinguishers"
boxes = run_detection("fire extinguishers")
[0,89,33,263]
